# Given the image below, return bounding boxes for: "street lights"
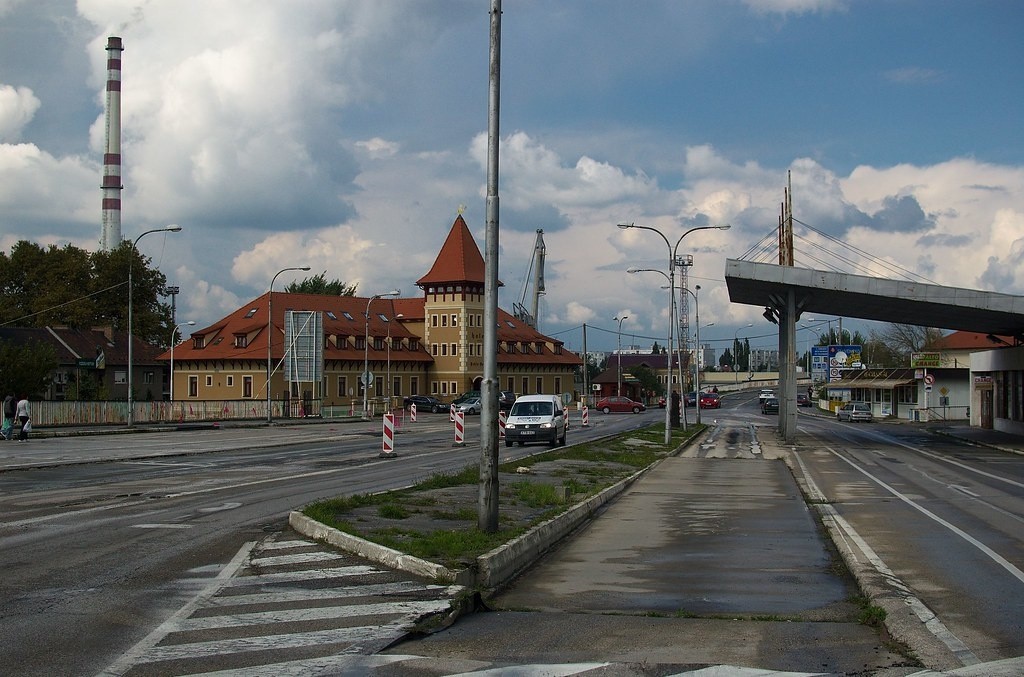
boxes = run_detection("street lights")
[735,324,753,384]
[613,316,628,396]
[836,326,851,344]
[388,313,404,413]
[128,225,182,426]
[364,290,400,421]
[617,223,731,432]
[808,318,831,343]
[170,321,197,421]
[269,265,312,421]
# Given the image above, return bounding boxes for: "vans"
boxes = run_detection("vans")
[504,395,566,447]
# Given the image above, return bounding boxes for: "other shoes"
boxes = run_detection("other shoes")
[19,439,27,442]
[0,431,7,439]
[5,438,13,440]
[26,438,30,442]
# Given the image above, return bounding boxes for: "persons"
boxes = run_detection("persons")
[16,394,32,443]
[640,388,651,407]
[705,385,718,394]
[807,386,813,399]
[0,391,17,440]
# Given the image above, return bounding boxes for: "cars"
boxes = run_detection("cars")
[451,391,481,415]
[405,396,450,414]
[700,393,721,408]
[837,403,871,421]
[683,391,705,407]
[659,398,666,409]
[596,396,646,414]
[797,393,812,407]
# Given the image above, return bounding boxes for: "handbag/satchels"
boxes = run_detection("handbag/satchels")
[1,418,9,430]
[23,419,32,432]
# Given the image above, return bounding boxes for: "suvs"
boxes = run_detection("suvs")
[499,391,515,408]
[759,390,779,415]
